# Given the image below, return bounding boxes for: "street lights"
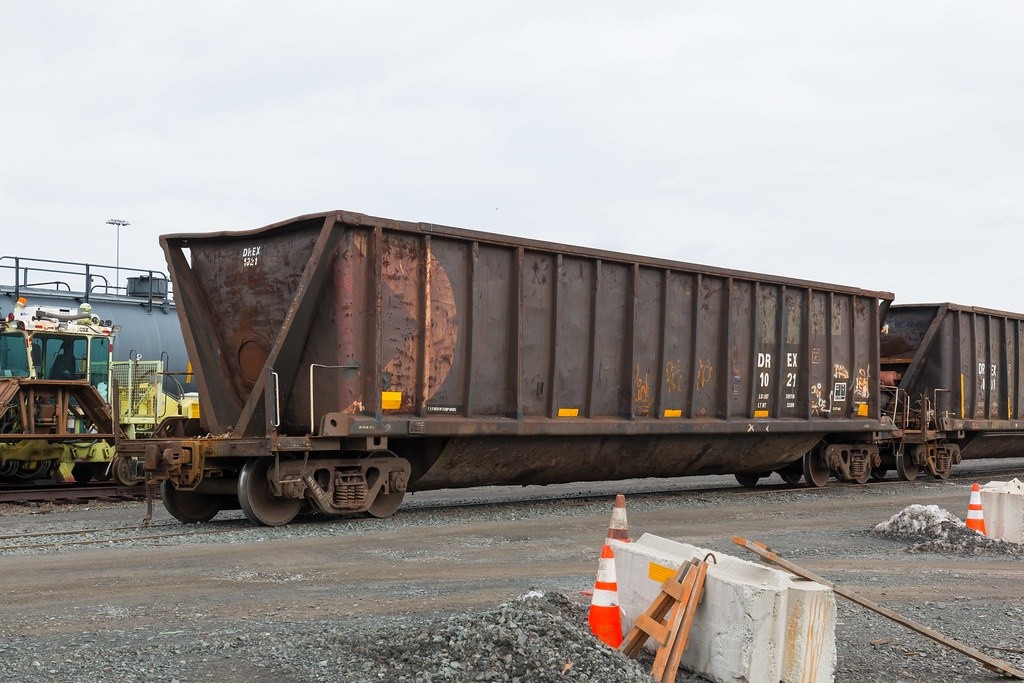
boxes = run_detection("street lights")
[105,218,131,295]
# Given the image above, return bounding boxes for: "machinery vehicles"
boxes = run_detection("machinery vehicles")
[0,295,204,488]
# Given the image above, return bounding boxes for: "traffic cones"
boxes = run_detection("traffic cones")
[604,493,633,545]
[586,542,624,651]
[965,482,987,537]
